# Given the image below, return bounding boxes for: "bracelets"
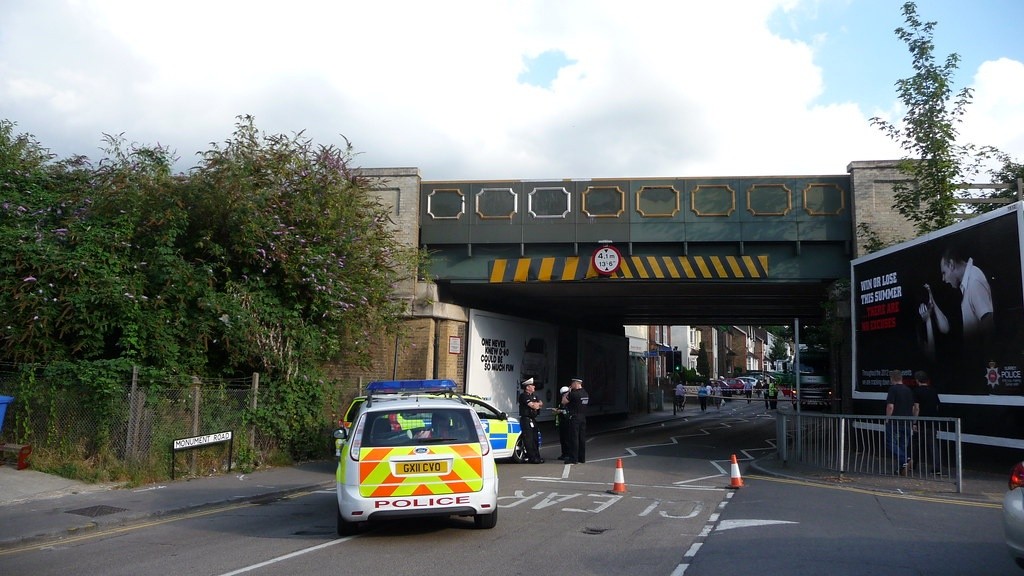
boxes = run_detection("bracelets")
[913,423,917,425]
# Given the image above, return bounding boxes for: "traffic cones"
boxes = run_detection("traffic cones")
[607,459,632,496]
[724,453,745,490]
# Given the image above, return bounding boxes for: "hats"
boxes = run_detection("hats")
[560,386,570,394]
[522,378,534,386]
[770,376,775,380]
[572,376,583,382]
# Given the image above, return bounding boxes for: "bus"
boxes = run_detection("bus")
[789,348,836,414]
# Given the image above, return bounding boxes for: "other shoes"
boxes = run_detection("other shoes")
[907,457,913,469]
[558,455,565,459]
[565,460,578,464]
[529,458,544,464]
[900,467,908,476]
[578,459,585,463]
[930,469,941,474]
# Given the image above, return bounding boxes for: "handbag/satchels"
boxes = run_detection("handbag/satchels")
[721,399,726,406]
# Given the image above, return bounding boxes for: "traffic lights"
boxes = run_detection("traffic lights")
[672,349,682,373]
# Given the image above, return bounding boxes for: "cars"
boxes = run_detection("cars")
[331,381,500,534]
[997,461,1024,573]
[333,392,541,460]
[707,373,774,401]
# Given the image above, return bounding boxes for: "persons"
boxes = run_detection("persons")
[415,411,451,438]
[769,379,778,410]
[756,380,762,397]
[697,382,716,412]
[564,378,589,464]
[674,381,686,407]
[886,370,944,478]
[744,380,752,404]
[762,380,769,409]
[711,382,722,409]
[915,240,996,360]
[518,377,543,463]
[557,386,571,461]
